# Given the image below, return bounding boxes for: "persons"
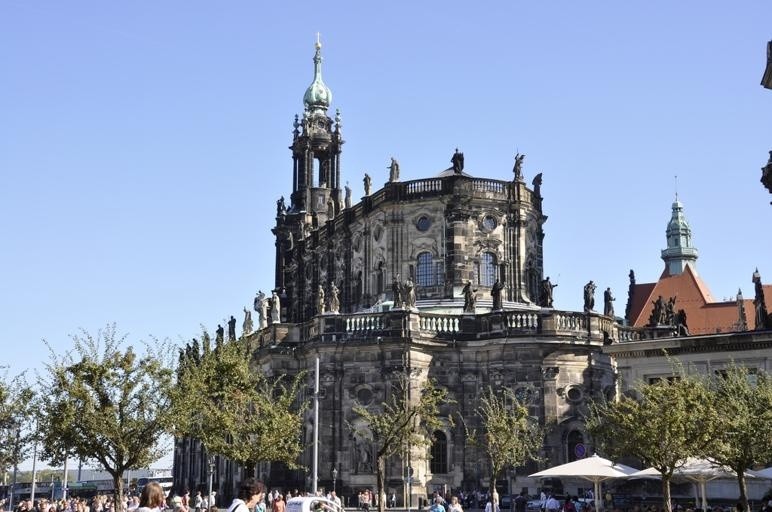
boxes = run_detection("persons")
[582,279,595,312]
[178,290,281,366]
[451,148,465,174]
[512,152,525,182]
[403,276,417,308]
[490,278,505,311]
[462,281,478,313]
[277,158,404,241]
[538,274,559,308]
[0,476,772,511]
[316,285,325,314]
[650,295,689,326]
[329,282,340,314]
[531,172,543,186]
[604,286,617,319]
[392,275,402,307]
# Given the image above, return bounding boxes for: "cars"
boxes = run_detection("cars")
[502,495,511,509]
[528,495,568,511]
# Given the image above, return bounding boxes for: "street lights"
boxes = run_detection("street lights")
[331,468,338,491]
[205,453,217,511]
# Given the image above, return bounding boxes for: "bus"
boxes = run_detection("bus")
[137,476,173,494]
[7,481,56,503]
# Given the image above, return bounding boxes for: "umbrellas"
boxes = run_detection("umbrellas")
[743,464,771,480]
[527,452,647,511]
[625,452,758,512]
